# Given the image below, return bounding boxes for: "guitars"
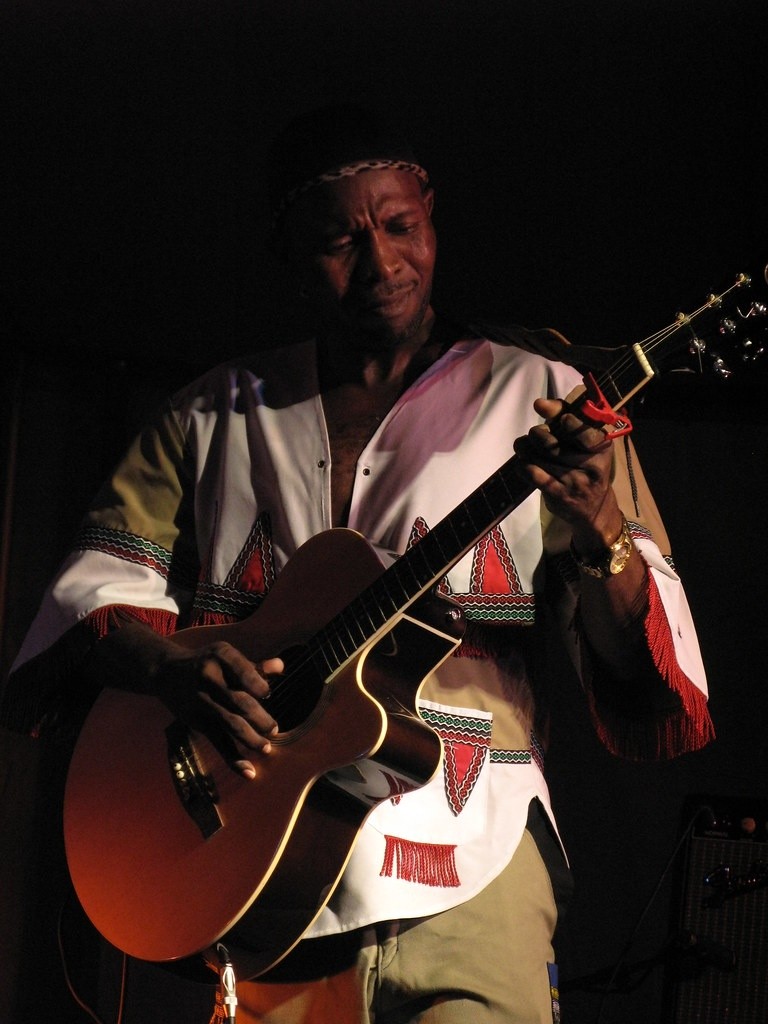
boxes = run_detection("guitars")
[62,264,766,978]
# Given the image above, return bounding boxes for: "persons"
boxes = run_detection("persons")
[0,102,716,1024]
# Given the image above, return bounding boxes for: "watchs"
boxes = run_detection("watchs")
[569,512,633,578]
